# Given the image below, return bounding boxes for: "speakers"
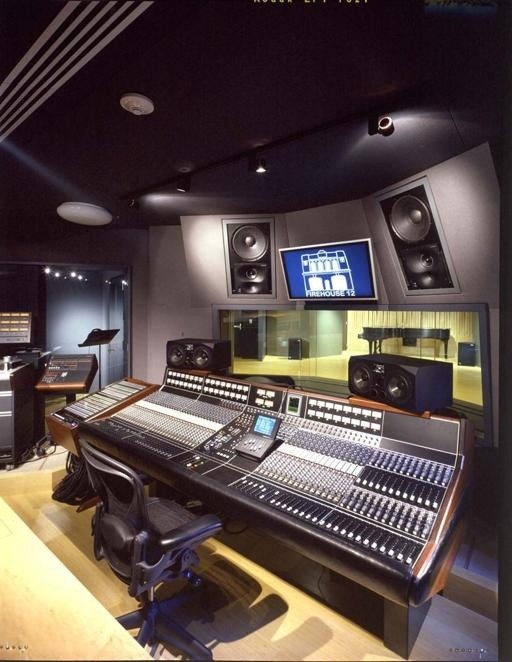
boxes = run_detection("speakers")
[376,175,462,297]
[221,218,276,301]
[165,338,231,371]
[288,337,302,360]
[459,342,477,367]
[347,353,453,413]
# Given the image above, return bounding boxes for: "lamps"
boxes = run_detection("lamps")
[175,173,188,191]
[251,153,265,174]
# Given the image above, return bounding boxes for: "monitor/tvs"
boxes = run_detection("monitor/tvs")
[278,238,379,310]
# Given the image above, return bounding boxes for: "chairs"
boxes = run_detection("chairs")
[77,438,223,660]
